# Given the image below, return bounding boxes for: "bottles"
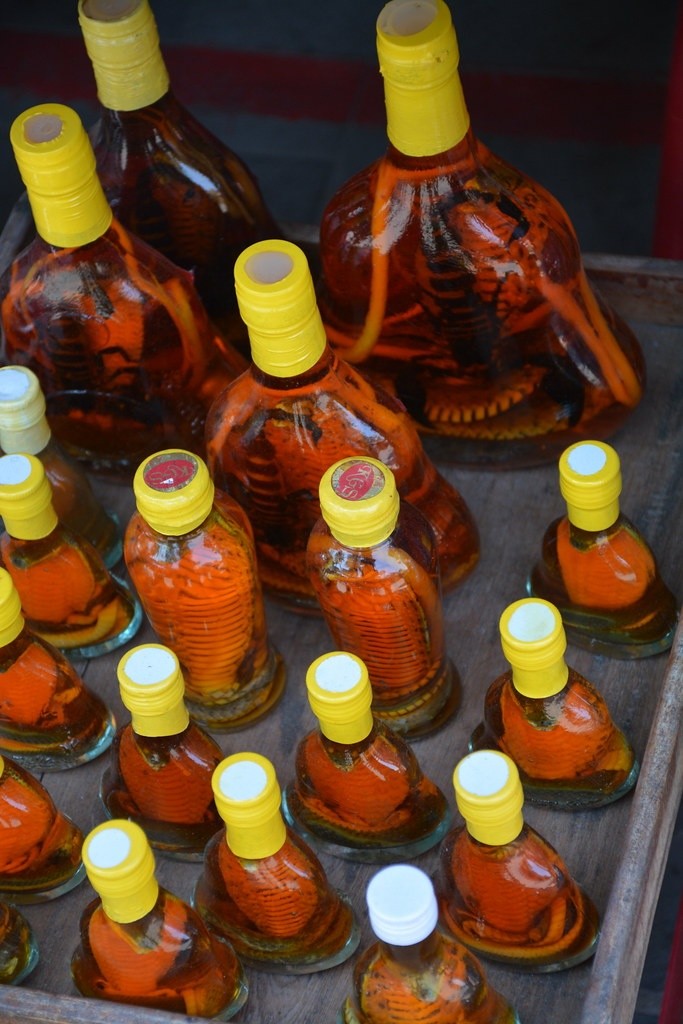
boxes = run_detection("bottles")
[70,0,278,348]
[317,0,645,470]
[0,365,674,1022]
[204,238,479,617]
[1,104,244,482]
